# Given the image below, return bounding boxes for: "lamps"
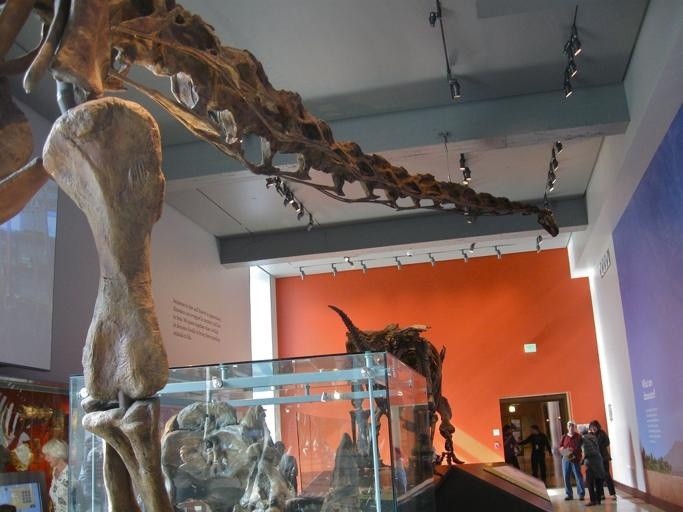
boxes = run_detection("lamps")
[458,153,472,185]
[561,25,581,98]
[452,81,462,99]
[548,139,563,192]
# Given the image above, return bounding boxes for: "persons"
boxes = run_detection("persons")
[558,420,617,507]
[503,424,553,482]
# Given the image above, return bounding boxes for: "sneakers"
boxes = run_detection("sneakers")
[564,494,617,506]
